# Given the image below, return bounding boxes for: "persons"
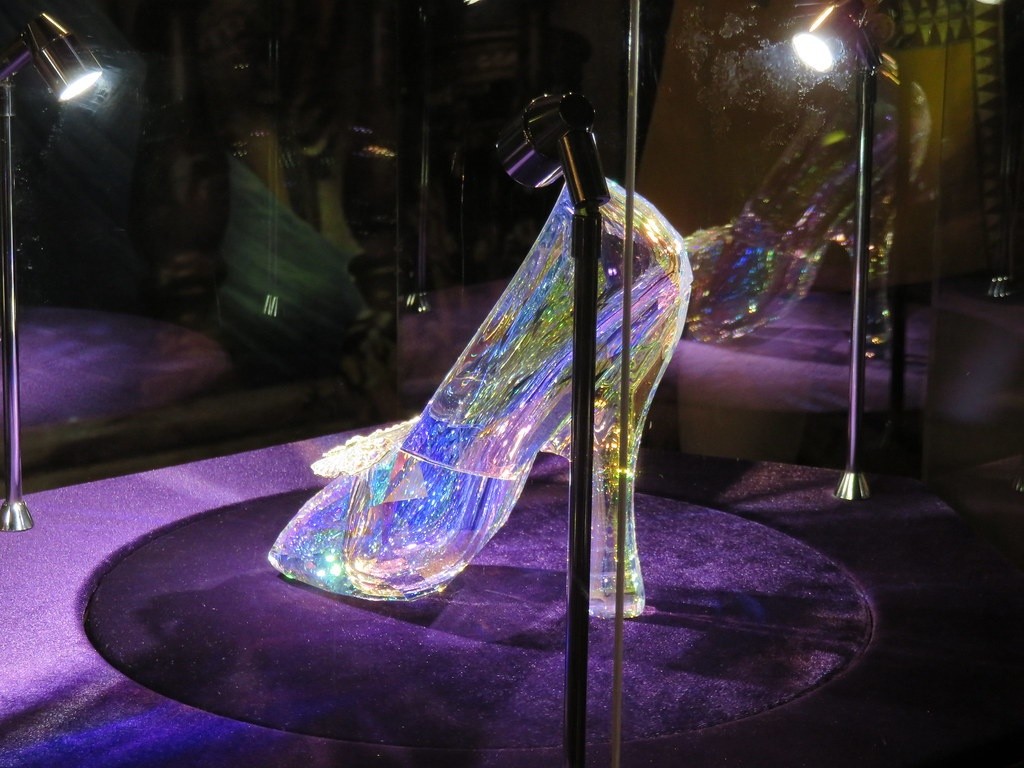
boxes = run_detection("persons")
[124,1,596,324]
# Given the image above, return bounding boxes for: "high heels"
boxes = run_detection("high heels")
[678,49,932,358]
[267,159,697,618]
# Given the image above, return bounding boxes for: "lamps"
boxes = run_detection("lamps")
[793,1,879,500]
[497,92,611,767]
[0,11,104,532]
